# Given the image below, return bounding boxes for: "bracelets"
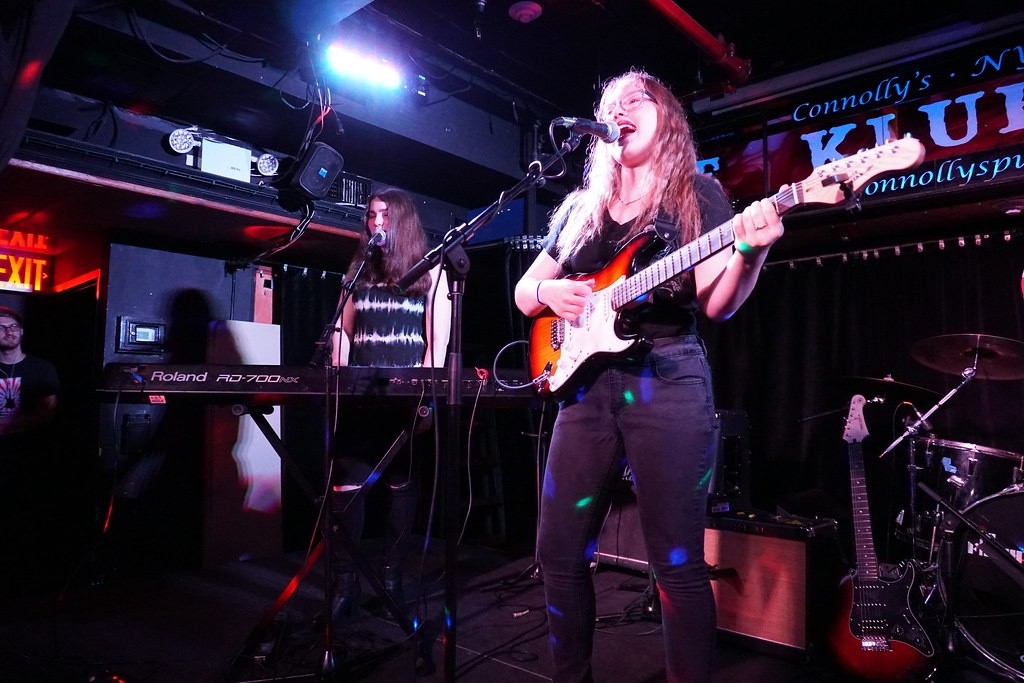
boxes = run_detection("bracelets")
[734,241,753,254]
[537,280,546,305]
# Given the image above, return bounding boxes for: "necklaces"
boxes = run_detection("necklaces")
[618,194,641,206]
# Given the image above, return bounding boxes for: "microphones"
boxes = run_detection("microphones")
[709,567,736,579]
[913,407,933,431]
[368,229,387,246]
[554,116,620,143]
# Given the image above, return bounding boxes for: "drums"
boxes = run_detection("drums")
[914,433,1024,502]
[934,483,1024,683]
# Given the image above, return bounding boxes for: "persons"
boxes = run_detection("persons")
[0,304,60,436]
[514,74,784,683]
[309,186,451,628]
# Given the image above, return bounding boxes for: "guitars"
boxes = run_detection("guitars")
[825,394,940,683]
[525,130,928,402]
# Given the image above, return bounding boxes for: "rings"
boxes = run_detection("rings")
[754,223,767,229]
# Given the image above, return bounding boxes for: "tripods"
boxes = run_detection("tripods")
[238,251,401,683]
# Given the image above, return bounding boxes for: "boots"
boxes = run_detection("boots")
[312,490,362,626]
[379,482,415,619]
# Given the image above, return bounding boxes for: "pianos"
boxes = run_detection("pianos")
[92,360,540,682]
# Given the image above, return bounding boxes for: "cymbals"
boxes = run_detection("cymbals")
[907,332,1024,381]
[842,374,945,401]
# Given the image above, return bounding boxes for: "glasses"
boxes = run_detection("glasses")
[598,89,658,121]
[0,324,21,334]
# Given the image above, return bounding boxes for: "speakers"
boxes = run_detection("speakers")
[292,142,344,199]
[706,411,751,500]
[702,513,839,662]
[589,464,649,575]
